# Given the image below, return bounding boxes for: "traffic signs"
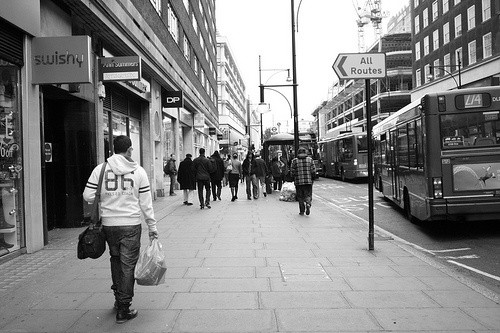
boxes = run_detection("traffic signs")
[332,53,387,82]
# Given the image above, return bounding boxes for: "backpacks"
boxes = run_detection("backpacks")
[164,160,171,175]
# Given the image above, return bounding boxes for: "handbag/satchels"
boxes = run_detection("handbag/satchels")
[227,164,232,171]
[134,238,167,287]
[77,227,106,260]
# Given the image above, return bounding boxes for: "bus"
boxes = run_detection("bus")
[317,131,375,180]
[262,132,323,178]
[371,86,500,223]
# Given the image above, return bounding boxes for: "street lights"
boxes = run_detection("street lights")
[354,0,387,250]
[258,55,292,148]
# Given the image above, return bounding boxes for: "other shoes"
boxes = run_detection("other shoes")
[254,196,257,199]
[264,192,266,197]
[169,193,176,196]
[184,201,187,205]
[117,309,136,323]
[187,203,192,205]
[217,196,221,201]
[206,204,211,209]
[231,198,235,202]
[299,211,304,215]
[248,196,251,200]
[201,206,204,210]
[235,196,238,199]
[306,205,310,216]
[213,197,217,201]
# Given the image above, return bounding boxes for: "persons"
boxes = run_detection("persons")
[82,135,158,322]
[177,154,196,205]
[242,152,258,200]
[254,153,268,198]
[269,156,288,192]
[225,153,242,202]
[290,148,316,215]
[221,153,232,187]
[192,148,213,209]
[169,153,178,196]
[210,151,224,201]
